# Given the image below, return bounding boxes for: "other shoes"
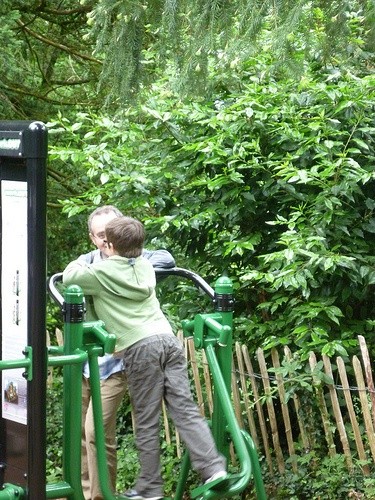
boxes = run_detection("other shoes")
[202,471,227,486]
[122,489,165,499]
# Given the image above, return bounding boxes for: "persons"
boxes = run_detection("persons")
[63,216,226,500]
[81,206,175,500]
